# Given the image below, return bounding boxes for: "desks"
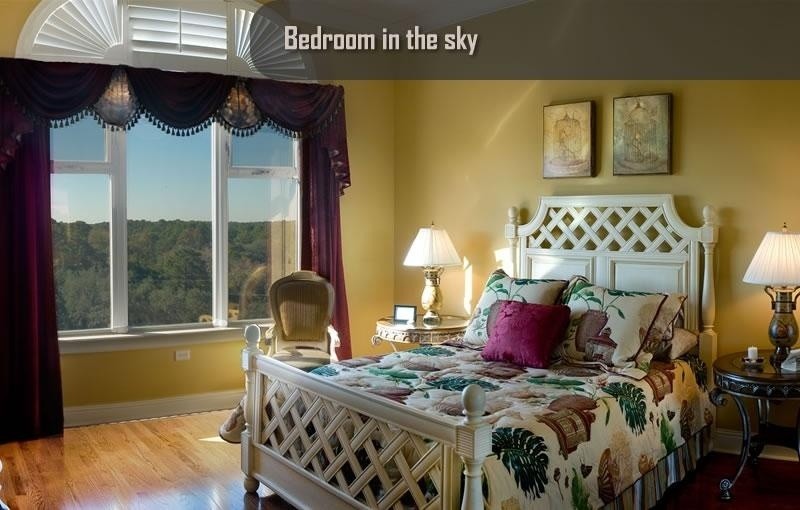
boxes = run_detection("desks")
[367,313,469,357]
[707,347,800,503]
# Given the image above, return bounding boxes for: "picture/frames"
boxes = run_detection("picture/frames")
[393,305,417,323]
[611,92,674,176]
[540,99,596,179]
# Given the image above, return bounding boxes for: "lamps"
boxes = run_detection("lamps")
[740,220,800,366]
[401,219,463,323]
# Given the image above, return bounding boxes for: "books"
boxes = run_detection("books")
[780,349,800,372]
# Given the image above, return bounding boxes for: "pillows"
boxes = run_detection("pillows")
[462,266,570,349]
[656,321,701,364]
[551,274,689,382]
[480,298,572,371]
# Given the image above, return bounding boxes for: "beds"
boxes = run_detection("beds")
[216,191,723,510]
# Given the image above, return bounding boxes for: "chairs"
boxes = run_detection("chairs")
[260,267,343,373]
